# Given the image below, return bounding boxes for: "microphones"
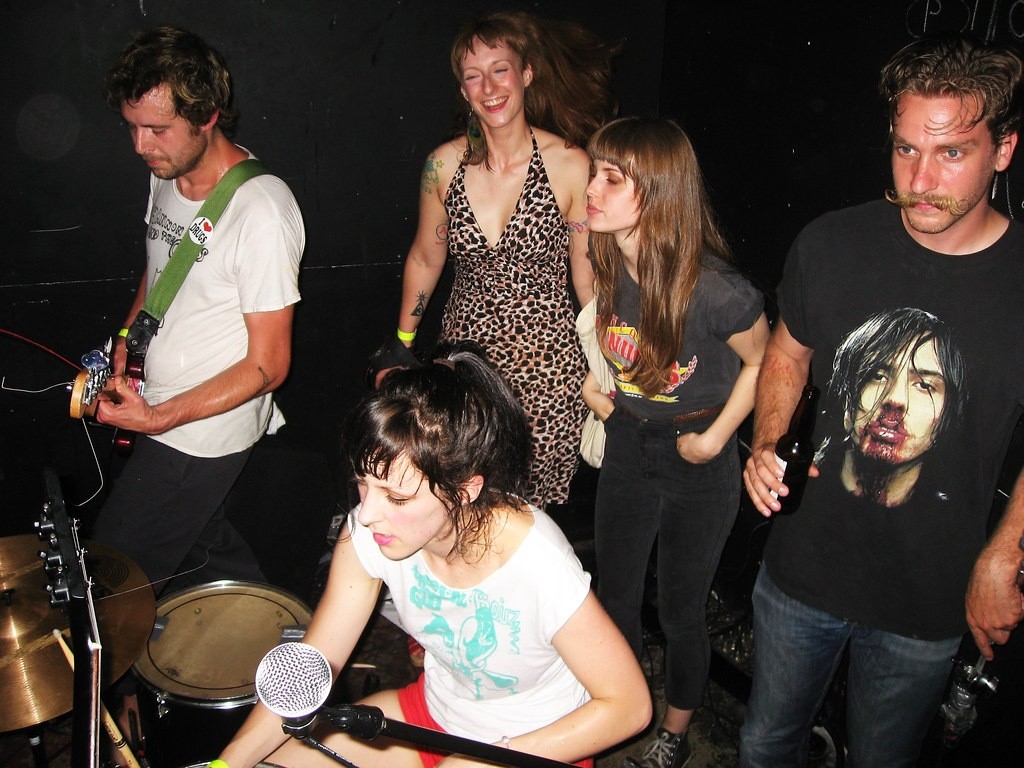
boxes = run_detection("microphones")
[255,641,333,745]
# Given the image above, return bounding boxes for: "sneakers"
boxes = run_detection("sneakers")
[636,728,693,768]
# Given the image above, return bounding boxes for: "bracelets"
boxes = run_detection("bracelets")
[205,760,228,767]
[118,328,129,337]
[397,328,418,342]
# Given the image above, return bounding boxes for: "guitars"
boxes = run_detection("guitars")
[64,336,147,459]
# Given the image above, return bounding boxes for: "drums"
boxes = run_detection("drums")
[131,578,315,768]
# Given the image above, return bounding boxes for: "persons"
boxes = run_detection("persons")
[733,37,1023,766]
[578,113,772,767]
[824,306,971,511]
[395,9,598,518]
[189,342,654,767]
[77,20,305,600]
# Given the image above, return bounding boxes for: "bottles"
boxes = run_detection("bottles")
[763,383,821,516]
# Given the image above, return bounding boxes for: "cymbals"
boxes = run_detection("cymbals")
[0,535,158,736]
